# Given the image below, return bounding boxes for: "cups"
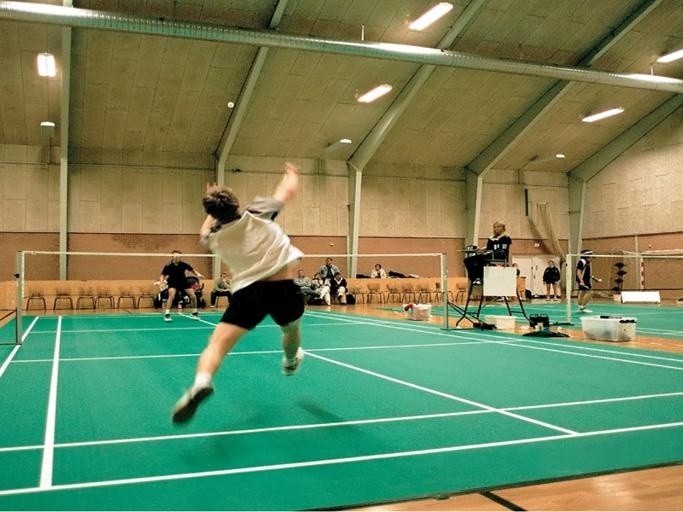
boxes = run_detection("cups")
[537,322,544,331]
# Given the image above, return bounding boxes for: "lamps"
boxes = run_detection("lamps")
[356,83,393,105]
[406,1,455,33]
[580,103,626,129]
[655,42,682,70]
[515,153,567,188]
[315,137,352,175]
[34,51,56,79]
[37,120,58,164]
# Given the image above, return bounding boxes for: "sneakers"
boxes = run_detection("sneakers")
[546,298,559,301]
[164,314,172,321]
[191,313,199,320]
[577,306,593,313]
[171,381,213,422]
[283,346,305,374]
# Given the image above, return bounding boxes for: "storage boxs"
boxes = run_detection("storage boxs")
[484,313,516,332]
[400,302,432,322]
[578,312,639,346]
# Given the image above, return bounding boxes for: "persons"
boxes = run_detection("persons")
[541,259,561,302]
[169,160,311,426]
[574,249,601,312]
[461,220,512,286]
[153,257,235,318]
[369,263,387,279]
[291,257,350,306]
[157,250,207,323]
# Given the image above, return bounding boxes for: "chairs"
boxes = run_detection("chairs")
[472,245,515,287]
